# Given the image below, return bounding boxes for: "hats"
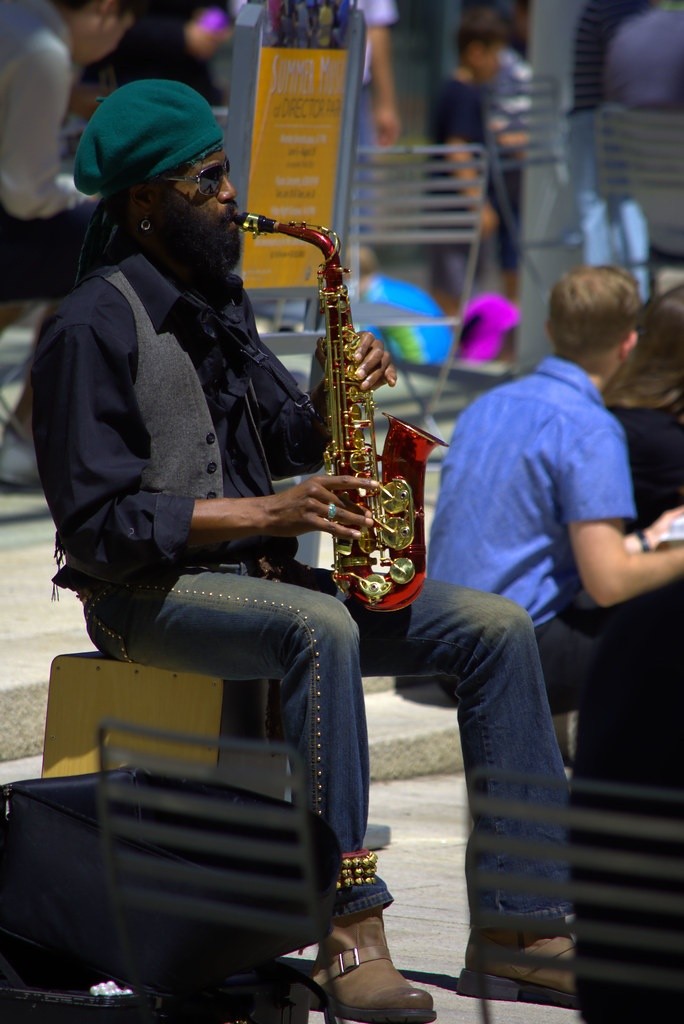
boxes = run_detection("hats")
[74,79,224,200]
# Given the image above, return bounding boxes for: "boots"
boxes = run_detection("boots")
[462,920,582,1010]
[309,905,437,1024]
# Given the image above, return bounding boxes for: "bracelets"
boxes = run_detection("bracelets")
[636,530,650,554]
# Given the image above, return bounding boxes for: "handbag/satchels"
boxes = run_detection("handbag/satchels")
[0,764,345,995]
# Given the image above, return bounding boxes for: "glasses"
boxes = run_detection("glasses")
[145,159,230,196]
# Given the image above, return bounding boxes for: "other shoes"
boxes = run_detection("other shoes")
[0,432,43,488]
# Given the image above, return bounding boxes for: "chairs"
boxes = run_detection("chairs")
[476,72,594,289]
[597,105,684,267]
[346,144,492,444]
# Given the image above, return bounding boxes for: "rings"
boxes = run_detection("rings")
[328,503,335,520]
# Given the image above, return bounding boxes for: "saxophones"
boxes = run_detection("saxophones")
[230,209,452,612]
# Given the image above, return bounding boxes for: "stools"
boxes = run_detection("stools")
[43,654,279,783]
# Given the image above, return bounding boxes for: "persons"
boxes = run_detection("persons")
[32,78,586,1024]
[1,0,684,792]
